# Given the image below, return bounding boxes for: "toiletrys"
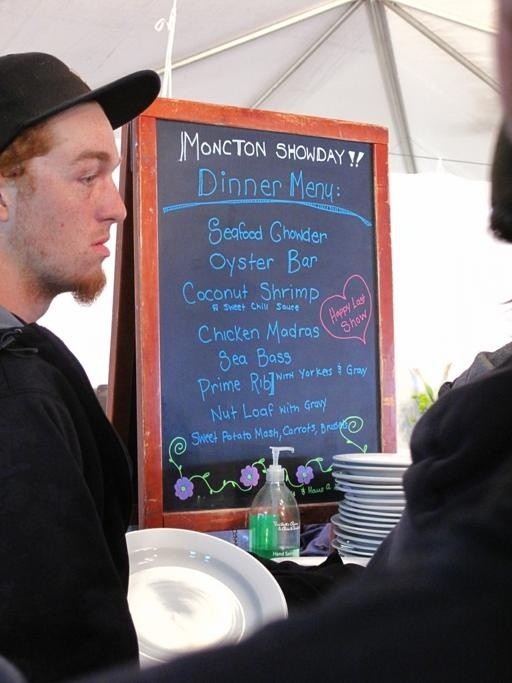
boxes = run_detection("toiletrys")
[246,444,304,561]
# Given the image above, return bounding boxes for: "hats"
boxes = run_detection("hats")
[0,53,161,157]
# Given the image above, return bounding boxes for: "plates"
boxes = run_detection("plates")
[329,452,413,558]
[124,528,290,671]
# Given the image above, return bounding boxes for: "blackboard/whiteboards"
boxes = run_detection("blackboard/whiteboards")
[130,93,396,531]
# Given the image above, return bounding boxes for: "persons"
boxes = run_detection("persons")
[0,51,161,682]
[0,116,511,683]
